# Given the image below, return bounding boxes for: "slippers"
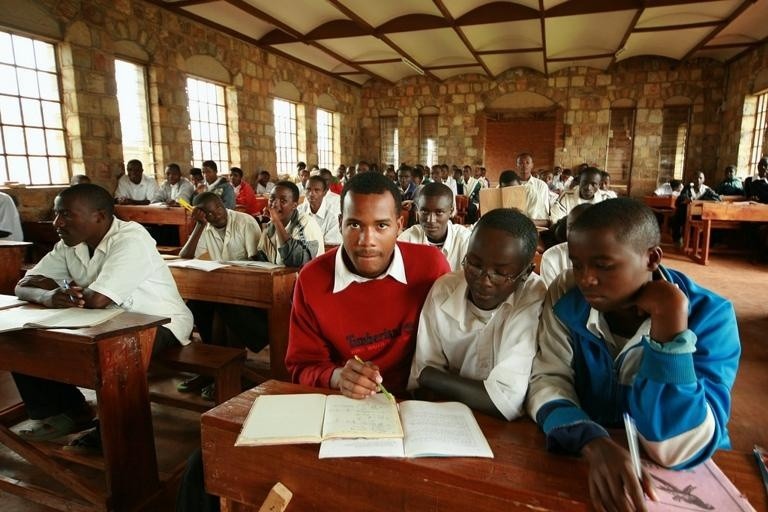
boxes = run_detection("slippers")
[177,376,199,391]
[201,383,215,400]
[19,413,96,440]
[62,428,101,455]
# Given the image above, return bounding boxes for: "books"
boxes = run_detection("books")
[168,254,281,273]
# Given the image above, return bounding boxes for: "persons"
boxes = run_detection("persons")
[2,154,768,511]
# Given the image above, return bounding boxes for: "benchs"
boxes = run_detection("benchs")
[720,196,748,202]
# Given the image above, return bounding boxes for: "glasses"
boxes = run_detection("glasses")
[460,255,531,287]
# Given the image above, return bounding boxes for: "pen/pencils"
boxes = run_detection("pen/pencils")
[354,355,396,405]
[63,279,75,302]
[623,412,647,500]
